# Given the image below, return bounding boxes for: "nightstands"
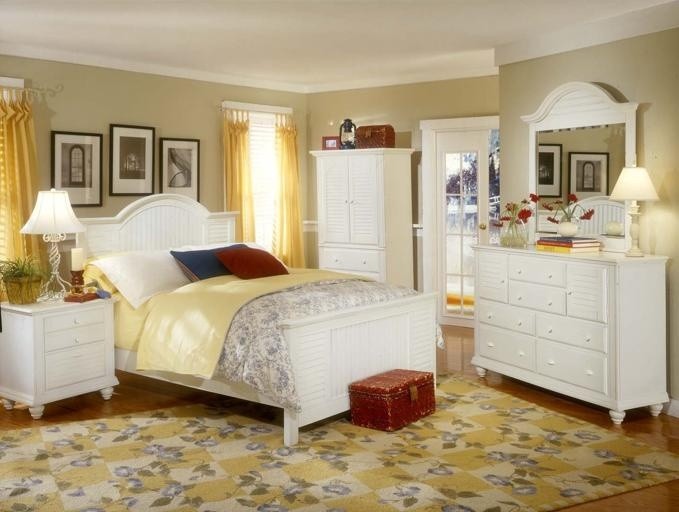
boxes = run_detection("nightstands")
[1,293,123,420]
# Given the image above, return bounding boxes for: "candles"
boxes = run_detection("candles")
[68,247,83,272]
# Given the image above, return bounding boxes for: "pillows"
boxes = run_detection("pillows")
[168,243,250,282]
[216,246,291,281]
[93,243,191,311]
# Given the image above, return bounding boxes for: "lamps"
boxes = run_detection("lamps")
[339,119,356,149]
[19,188,87,305]
[610,166,657,258]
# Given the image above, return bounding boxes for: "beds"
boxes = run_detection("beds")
[71,193,437,446]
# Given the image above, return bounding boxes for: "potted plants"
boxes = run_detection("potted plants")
[0,257,49,306]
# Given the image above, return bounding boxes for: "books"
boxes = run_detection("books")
[534,236,601,255]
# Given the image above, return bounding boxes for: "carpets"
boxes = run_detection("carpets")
[0,364,679,512]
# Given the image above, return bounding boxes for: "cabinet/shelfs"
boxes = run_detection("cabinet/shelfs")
[471,236,670,428]
[310,149,421,290]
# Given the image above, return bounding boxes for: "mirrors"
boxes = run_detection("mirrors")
[534,122,627,233]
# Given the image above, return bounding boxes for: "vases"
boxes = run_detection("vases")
[556,222,580,237]
[501,220,529,247]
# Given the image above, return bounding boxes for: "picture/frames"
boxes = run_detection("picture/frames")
[322,137,340,150]
[49,130,104,208]
[161,137,201,201]
[539,144,561,198]
[569,152,609,199]
[109,123,156,197]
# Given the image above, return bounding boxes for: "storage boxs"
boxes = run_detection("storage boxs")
[348,368,435,434]
[356,125,396,149]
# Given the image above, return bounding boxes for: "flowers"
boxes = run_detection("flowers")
[500,194,540,229]
[543,195,595,221]
